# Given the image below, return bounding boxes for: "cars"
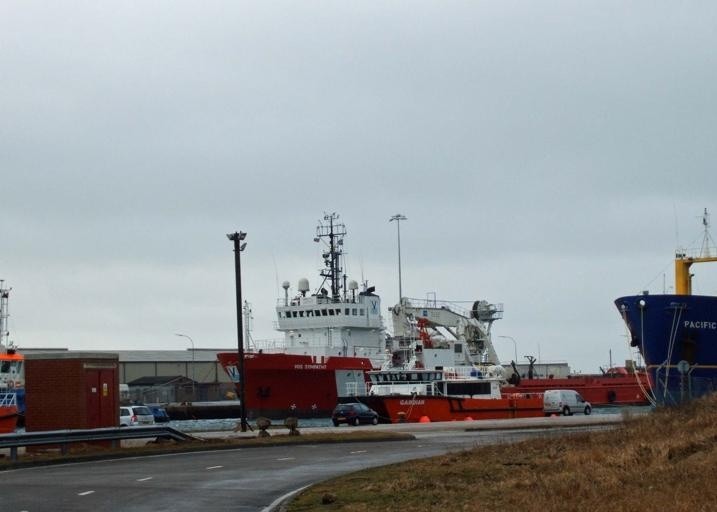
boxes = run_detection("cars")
[118,406,155,427]
[332,403,379,426]
